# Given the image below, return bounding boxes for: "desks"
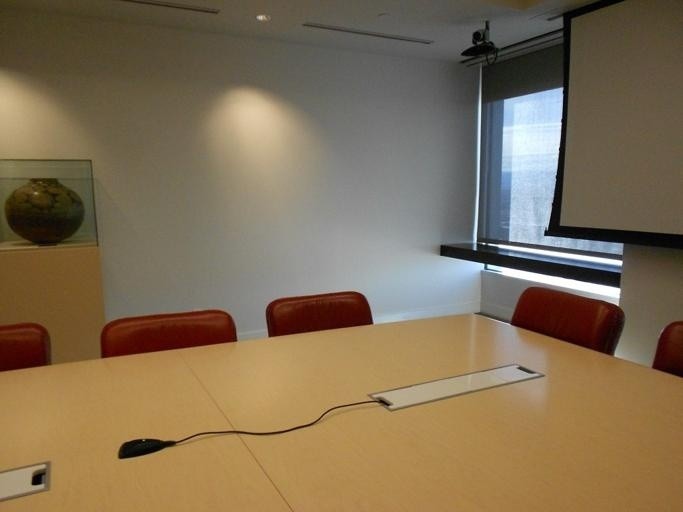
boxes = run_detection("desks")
[0,311,682,512]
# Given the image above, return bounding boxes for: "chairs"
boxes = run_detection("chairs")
[98,291,374,359]
[510,285,626,356]
